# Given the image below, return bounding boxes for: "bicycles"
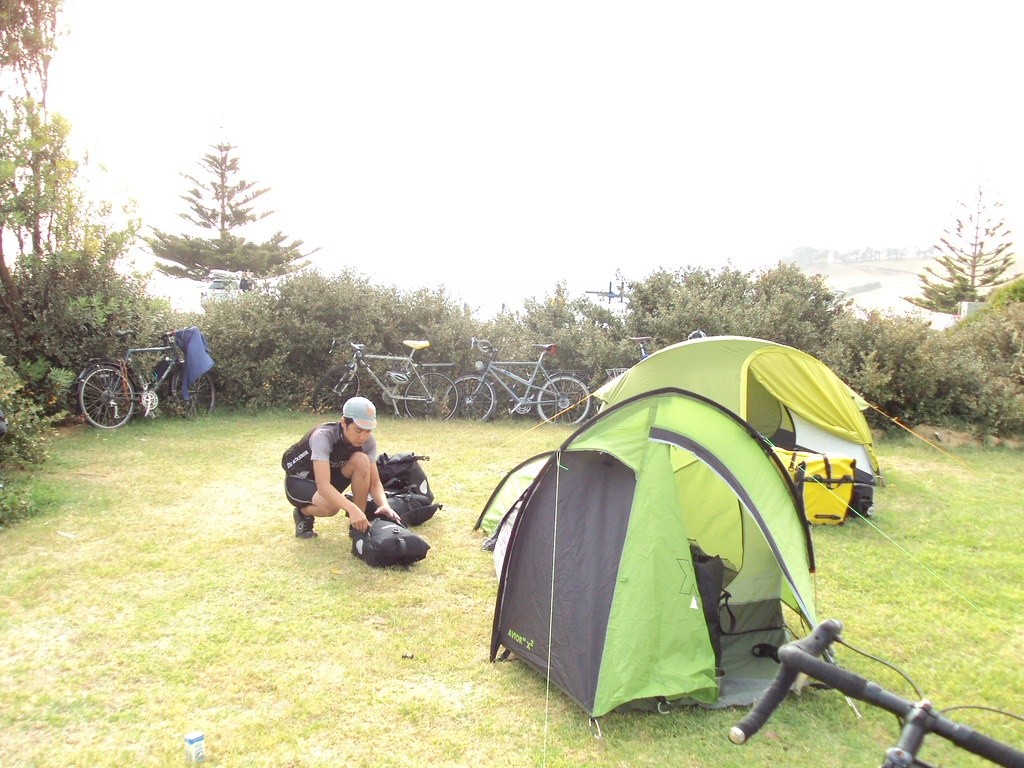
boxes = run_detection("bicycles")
[603,328,709,384]
[77,327,218,430]
[311,338,459,422]
[441,336,592,427]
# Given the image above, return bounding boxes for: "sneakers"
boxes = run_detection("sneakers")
[293,507,317,537]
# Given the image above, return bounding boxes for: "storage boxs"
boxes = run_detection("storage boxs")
[796,455,856,526]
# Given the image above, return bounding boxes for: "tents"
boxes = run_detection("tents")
[590,335,887,490]
[466,387,859,732]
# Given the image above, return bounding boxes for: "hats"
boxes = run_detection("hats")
[343,397,378,429]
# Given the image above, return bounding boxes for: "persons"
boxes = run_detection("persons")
[281,397,402,539]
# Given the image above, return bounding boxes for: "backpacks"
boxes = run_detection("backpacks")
[344,478,443,527]
[375,452,434,503]
[348,516,431,572]
